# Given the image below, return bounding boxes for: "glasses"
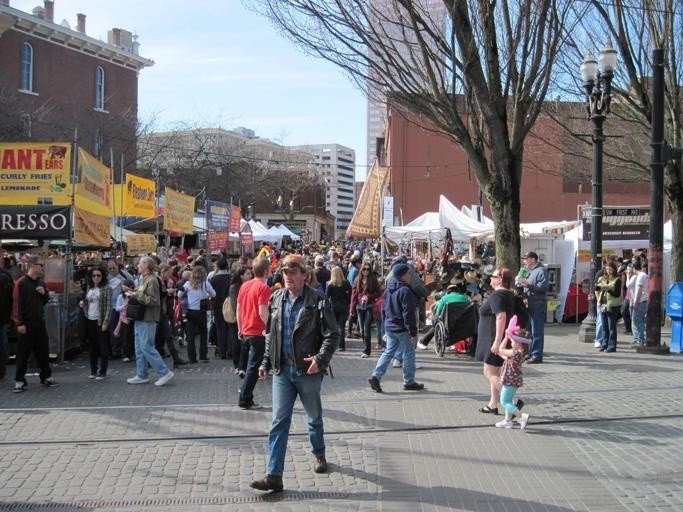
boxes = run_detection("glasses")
[34,263,45,268]
[361,267,371,271]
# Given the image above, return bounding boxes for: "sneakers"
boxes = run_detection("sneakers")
[153,370,175,386]
[495,419,514,429]
[182,312,189,323]
[524,354,543,364]
[86,335,246,381]
[248,474,283,492]
[624,330,646,345]
[337,329,386,359]
[594,341,601,348]
[392,358,402,368]
[246,401,262,410]
[313,454,328,473]
[126,374,150,385]
[404,380,425,390]
[516,412,530,431]
[40,377,60,386]
[598,345,616,352]
[12,380,29,393]
[367,374,382,392]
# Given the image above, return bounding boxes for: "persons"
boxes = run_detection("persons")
[593,253,648,352]
[249,254,340,491]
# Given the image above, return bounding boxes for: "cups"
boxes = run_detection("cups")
[520,268,527,278]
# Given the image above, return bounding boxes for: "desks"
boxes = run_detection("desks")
[564,287,589,324]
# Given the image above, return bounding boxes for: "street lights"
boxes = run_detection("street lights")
[575,33,616,346]
[192,161,225,191]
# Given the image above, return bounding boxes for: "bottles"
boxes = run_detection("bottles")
[361,295,367,310]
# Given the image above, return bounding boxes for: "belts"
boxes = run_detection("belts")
[281,357,295,366]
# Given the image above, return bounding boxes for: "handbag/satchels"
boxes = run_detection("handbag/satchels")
[511,294,530,330]
[199,297,215,311]
[600,303,613,314]
[125,297,146,321]
[221,295,238,324]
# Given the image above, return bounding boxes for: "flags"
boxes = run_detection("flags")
[345,158,391,239]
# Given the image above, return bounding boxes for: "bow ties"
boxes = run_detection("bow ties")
[416,340,429,350]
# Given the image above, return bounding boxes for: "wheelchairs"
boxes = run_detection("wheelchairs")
[430,300,479,358]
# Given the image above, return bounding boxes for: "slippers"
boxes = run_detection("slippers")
[516,398,525,411]
[478,405,498,415]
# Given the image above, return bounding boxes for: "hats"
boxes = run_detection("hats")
[464,269,476,283]
[519,251,538,260]
[280,253,307,274]
[392,263,409,280]
[449,273,466,285]
[315,254,325,264]
[483,263,495,276]
